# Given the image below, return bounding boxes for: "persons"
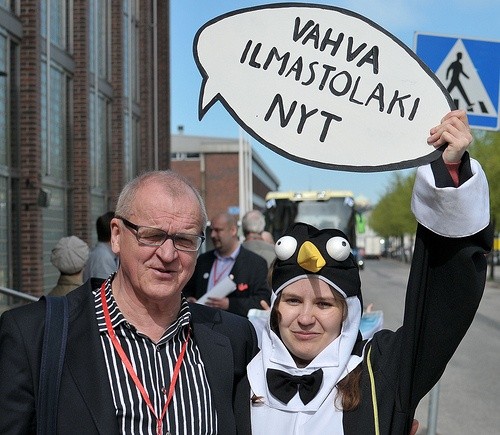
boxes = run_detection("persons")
[0,171,420,435]
[46,209,281,318]
[236,110,491,435]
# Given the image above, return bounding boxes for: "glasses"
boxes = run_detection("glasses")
[117,215,205,251]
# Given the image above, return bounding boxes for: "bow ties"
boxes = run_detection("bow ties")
[266,369,323,405]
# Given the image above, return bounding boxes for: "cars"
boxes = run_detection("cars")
[357,254,364,270]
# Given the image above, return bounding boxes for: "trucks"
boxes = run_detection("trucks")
[364,236,381,260]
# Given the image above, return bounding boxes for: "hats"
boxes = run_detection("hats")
[269,222,363,322]
[50,236,89,272]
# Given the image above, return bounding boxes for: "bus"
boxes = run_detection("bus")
[266,190,366,266]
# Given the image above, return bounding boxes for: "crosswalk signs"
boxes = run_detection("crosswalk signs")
[413,32,500,131]
